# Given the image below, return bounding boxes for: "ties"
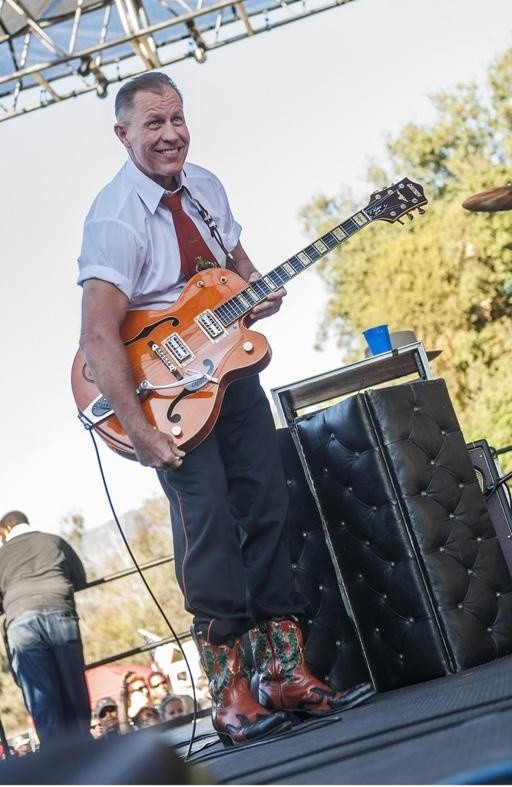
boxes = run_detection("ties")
[161,192,221,281]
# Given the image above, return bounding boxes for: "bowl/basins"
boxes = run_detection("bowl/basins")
[364,329,418,359]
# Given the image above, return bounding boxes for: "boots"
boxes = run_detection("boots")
[244,616,377,719]
[193,634,294,747]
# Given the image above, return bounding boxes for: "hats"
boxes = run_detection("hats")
[94,697,117,717]
[13,735,31,750]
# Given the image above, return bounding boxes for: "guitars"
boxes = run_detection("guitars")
[72,178,429,461]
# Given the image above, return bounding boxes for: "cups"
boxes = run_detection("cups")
[363,321,391,356]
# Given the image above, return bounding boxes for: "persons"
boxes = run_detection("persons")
[73,73,375,746]
[0,510,93,752]
[1,669,200,760]
